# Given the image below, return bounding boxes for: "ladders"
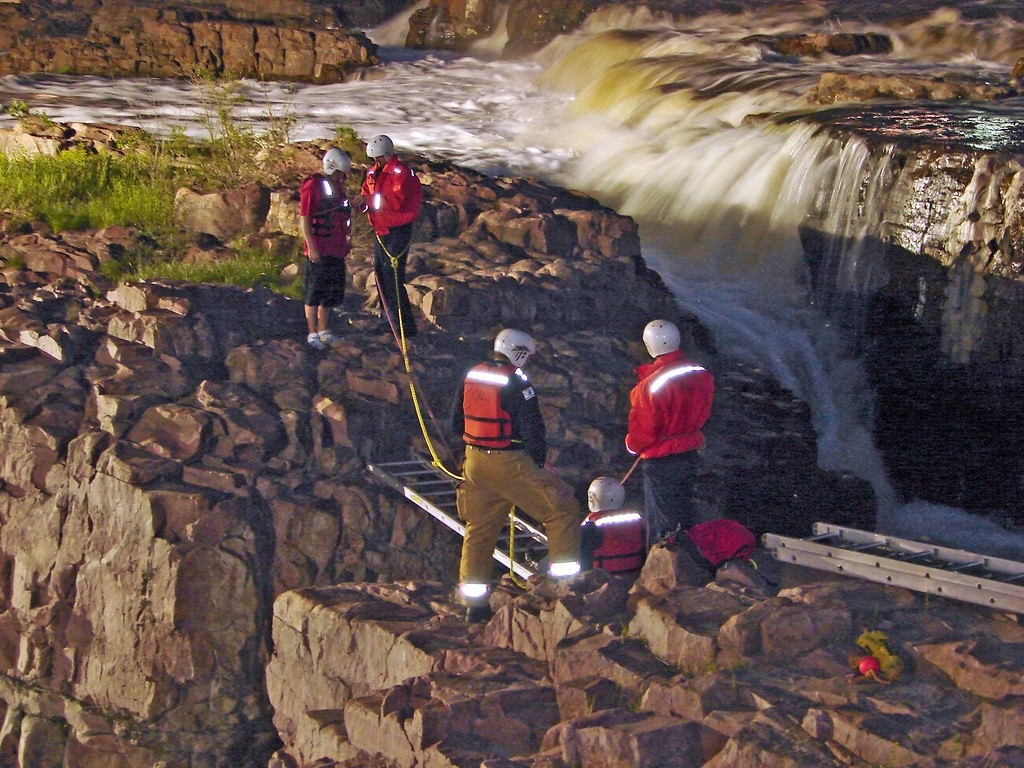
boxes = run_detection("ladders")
[365,453,548,581]
[760,521,1024,618]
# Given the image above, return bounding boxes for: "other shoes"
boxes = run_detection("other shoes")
[307,333,325,351]
[318,329,340,343]
[394,324,417,339]
[366,324,392,337]
[465,604,495,623]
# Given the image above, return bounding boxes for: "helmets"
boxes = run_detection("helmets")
[587,476,625,512]
[323,147,351,176]
[642,320,680,358]
[494,328,536,367]
[366,135,394,162]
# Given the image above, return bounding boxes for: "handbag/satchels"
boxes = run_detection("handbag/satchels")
[660,518,757,578]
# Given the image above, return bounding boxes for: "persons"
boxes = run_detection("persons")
[299,149,355,349]
[349,135,422,337]
[456,329,587,623]
[580,475,650,577]
[625,319,714,558]
